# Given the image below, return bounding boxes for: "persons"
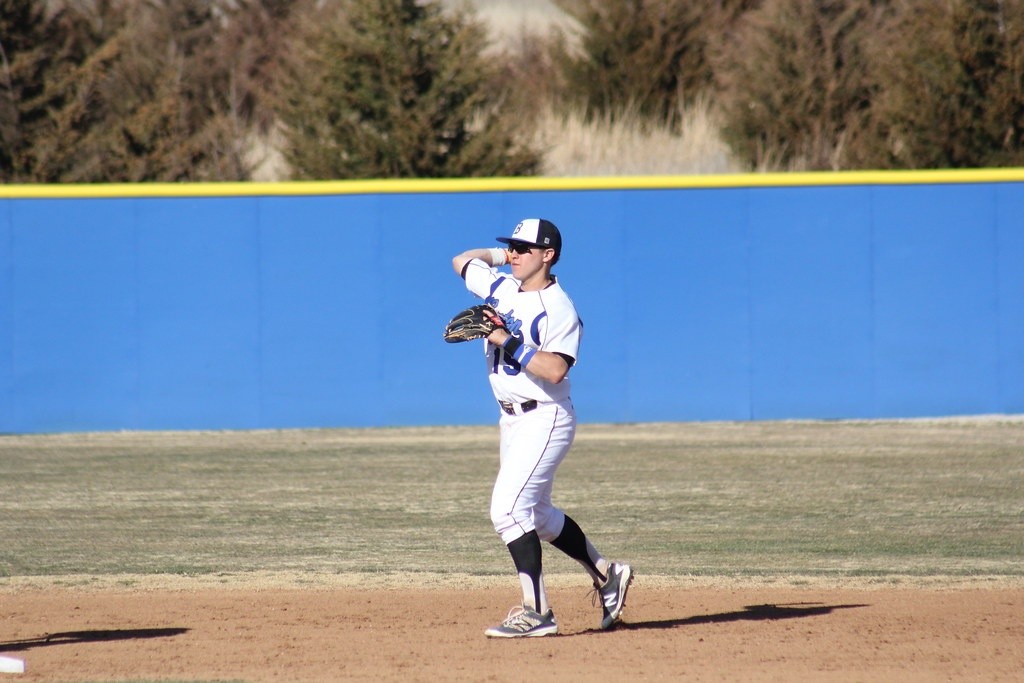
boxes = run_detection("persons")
[453,219,635,638]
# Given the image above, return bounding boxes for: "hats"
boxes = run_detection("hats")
[496,219,562,251]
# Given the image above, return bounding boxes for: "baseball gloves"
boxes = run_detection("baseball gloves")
[443,305,506,343]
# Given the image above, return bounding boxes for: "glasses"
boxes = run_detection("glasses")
[506,241,545,255]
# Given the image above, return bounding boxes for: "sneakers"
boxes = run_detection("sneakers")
[584,562,634,628]
[484,606,558,637]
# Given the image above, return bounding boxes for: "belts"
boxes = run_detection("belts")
[498,399,537,415]
